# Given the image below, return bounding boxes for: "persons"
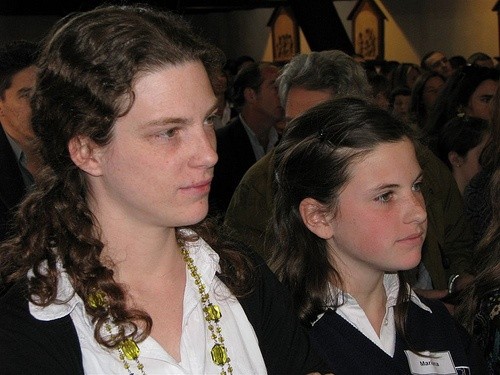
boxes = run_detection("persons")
[0,41,39,240]
[206,51,500,375]
[0,4,333,375]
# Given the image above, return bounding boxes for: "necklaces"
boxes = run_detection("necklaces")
[86,238,234,375]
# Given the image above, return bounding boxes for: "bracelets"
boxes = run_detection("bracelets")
[447,274,460,293]
